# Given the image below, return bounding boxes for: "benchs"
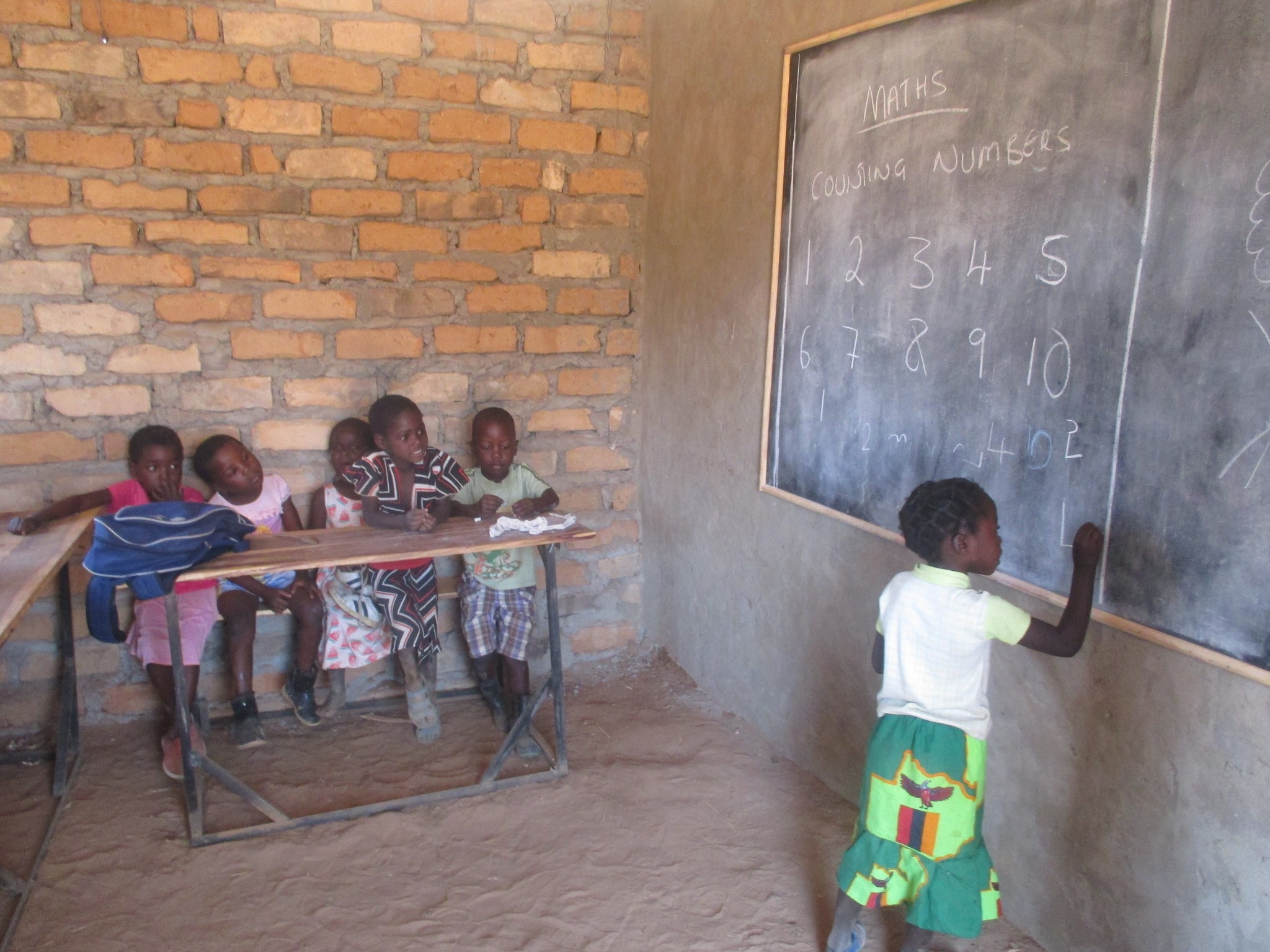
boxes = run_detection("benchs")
[194,576,486,728]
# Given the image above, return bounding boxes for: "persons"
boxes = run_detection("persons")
[193,434,325,751]
[449,407,560,759]
[332,394,470,745]
[824,479,1104,952]
[307,418,397,719]
[0,425,220,779]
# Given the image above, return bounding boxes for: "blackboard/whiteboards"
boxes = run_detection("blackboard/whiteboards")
[758,0,1270,686]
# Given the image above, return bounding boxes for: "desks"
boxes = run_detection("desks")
[116,508,596,849]
[0,507,106,952]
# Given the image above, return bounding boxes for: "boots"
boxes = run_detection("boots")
[280,660,322,726]
[231,691,266,749]
[472,670,513,732]
[508,694,540,758]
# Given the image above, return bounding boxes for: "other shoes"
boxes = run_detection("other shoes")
[826,921,866,952]
[190,714,206,756]
[160,732,185,779]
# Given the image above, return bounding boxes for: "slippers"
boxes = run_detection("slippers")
[404,673,438,728]
[415,718,440,744]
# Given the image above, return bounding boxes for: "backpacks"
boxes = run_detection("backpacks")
[85,500,258,644]
